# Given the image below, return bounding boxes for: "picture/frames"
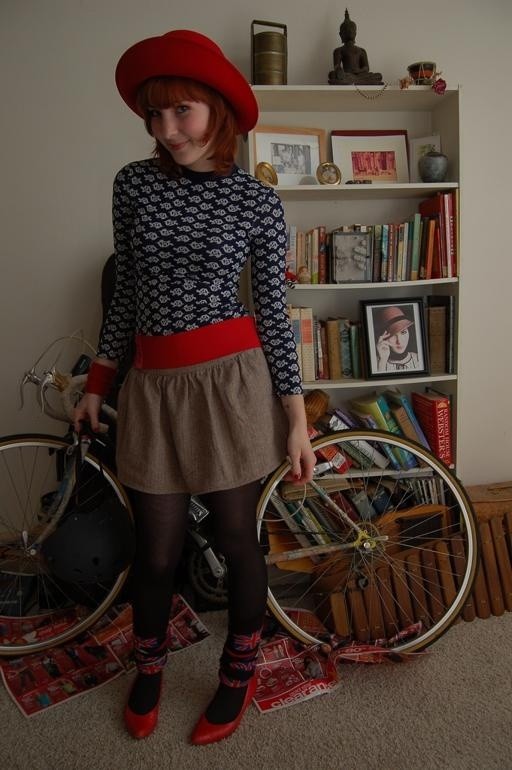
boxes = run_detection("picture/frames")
[357,297,430,380]
[251,125,327,185]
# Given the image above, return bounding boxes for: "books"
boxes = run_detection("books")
[285,191,455,285]
[289,296,453,382]
[270,387,456,563]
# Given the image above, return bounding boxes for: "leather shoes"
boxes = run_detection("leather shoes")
[192,678,259,745]
[124,675,163,740]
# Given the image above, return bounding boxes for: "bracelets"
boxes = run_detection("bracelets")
[81,361,118,398]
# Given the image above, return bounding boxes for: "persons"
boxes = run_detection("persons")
[327,8,383,84]
[71,30,318,747]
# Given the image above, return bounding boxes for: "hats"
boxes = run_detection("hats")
[116,30,259,135]
[377,308,412,338]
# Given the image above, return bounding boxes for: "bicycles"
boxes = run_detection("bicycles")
[0,327,479,659]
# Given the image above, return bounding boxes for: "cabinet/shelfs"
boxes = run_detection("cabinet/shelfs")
[238,83,472,487]
